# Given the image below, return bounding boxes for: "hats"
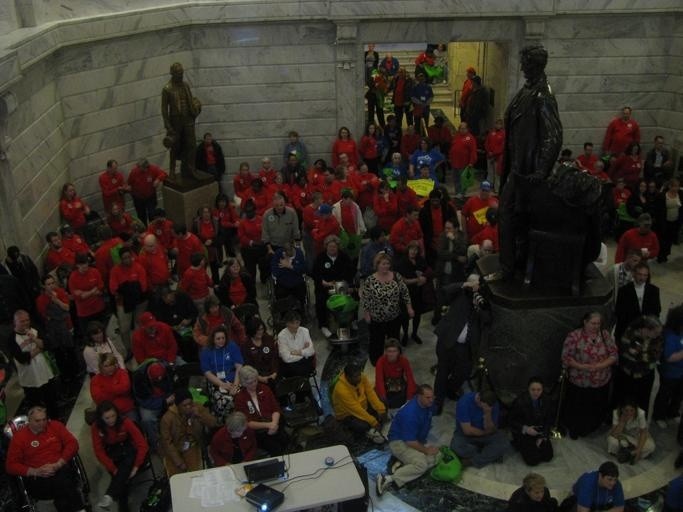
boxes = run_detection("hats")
[140,312,154,326]
[315,204,335,214]
[481,182,492,188]
[466,65,483,82]
[205,296,220,305]
[175,387,192,406]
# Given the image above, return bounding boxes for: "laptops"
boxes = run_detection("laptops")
[244,459,285,484]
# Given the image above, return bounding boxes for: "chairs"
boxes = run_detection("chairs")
[275,370,322,420]
[16,420,156,512]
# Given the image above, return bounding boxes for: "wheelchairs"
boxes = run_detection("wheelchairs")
[265,271,313,335]
[4,416,91,511]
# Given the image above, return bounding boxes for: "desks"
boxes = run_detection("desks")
[167,444,365,512]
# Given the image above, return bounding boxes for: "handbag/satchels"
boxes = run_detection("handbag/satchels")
[368,428,383,442]
[388,457,401,474]
[430,445,462,482]
[402,334,409,346]
[410,333,422,345]
[618,448,631,463]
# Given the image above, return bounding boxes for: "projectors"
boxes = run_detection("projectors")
[245,484,284,512]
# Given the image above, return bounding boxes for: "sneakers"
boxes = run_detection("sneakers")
[657,258,667,263]
[377,473,388,495]
[98,498,113,510]
[430,317,444,324]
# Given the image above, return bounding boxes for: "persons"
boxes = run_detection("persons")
[3,105,681,510]
[498,45,562,284]
[162,62,201,179]
[364,44,489,137]
